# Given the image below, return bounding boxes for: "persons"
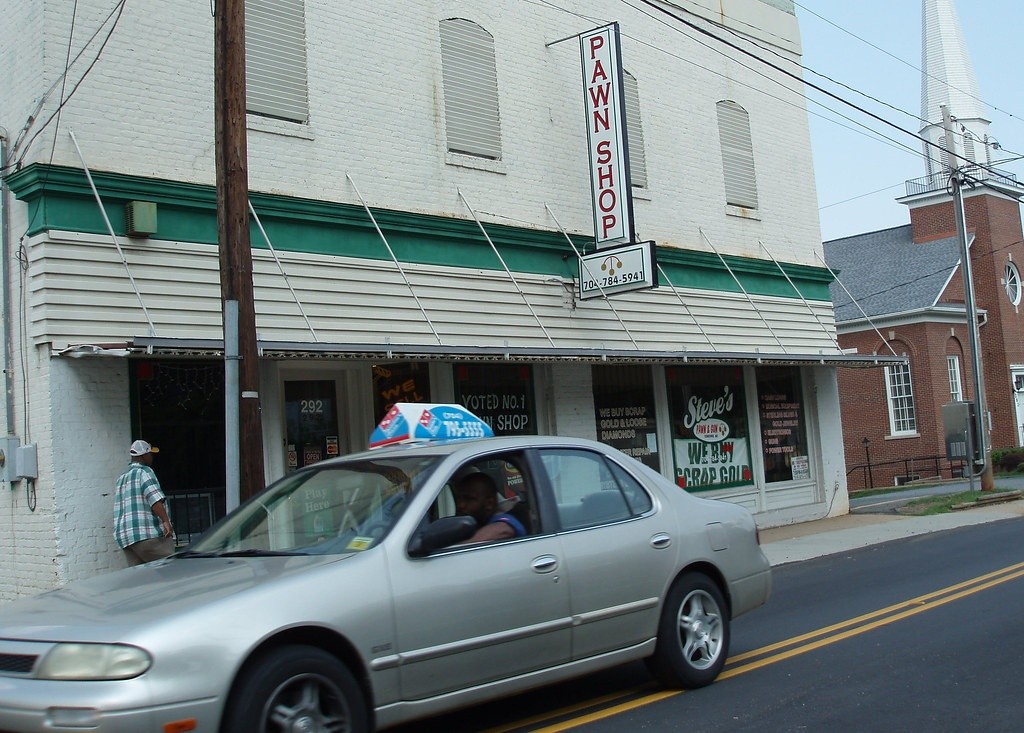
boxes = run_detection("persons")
[113,440,175,568]
[456,472,526,544]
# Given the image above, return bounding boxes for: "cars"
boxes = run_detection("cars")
[0,401,771,733]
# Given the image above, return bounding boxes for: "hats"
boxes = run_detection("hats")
[130,440,159,456]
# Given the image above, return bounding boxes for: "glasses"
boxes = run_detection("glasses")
[452,493,478,504]
